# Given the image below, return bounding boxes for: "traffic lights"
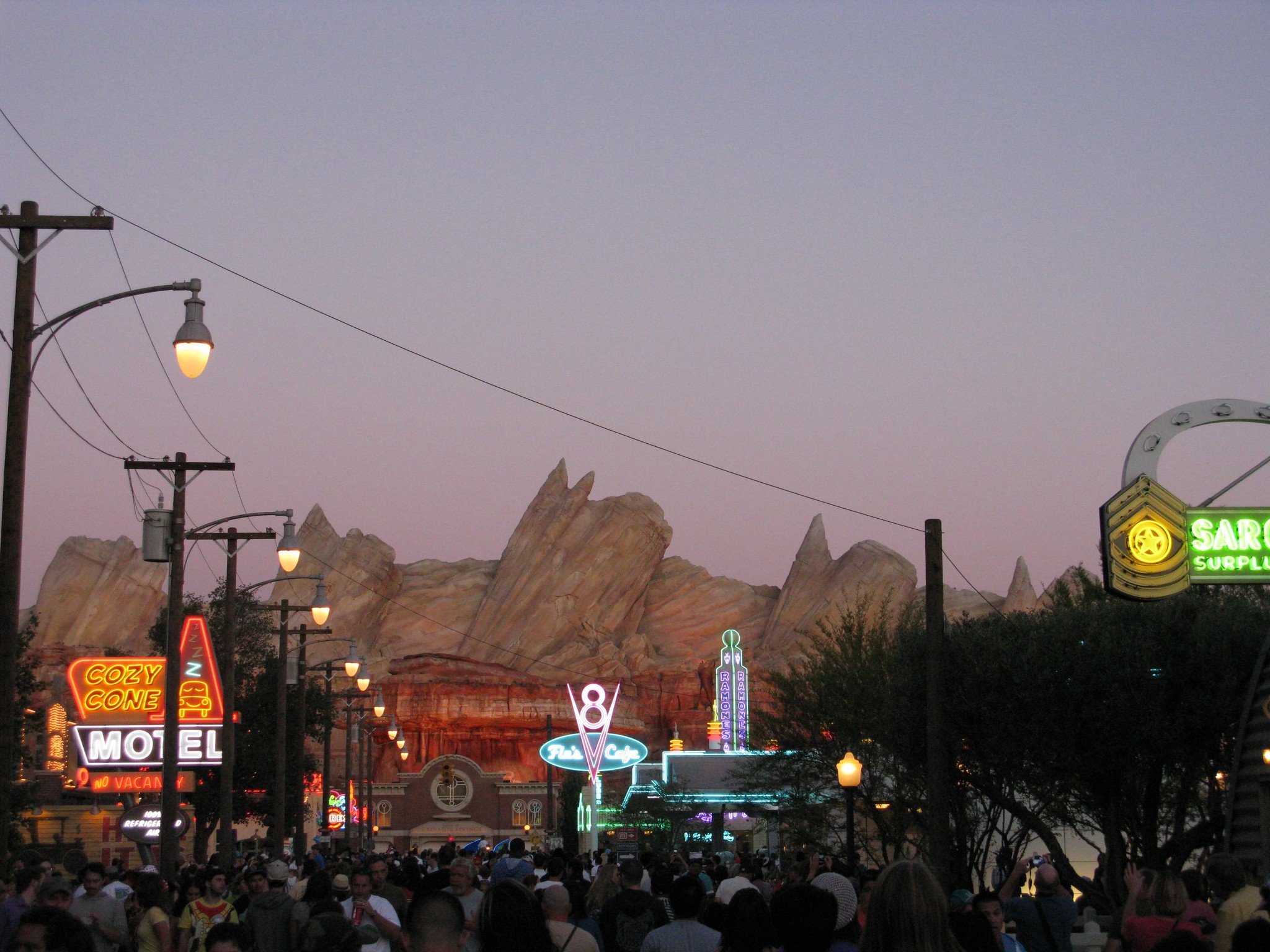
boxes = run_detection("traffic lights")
[443,764,450,785]
[525,825,530,835]
[373,826,378,836]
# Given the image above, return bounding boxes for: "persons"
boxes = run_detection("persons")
[1,822,1270,952]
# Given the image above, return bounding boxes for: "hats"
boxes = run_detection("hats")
[243,860,351,901]
[811,873,856,933]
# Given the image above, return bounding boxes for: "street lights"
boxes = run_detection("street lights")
[0,201,214,884]
[188,527,332,876]
[269,623,372,856]
[366,719,404,844]
[366,732,409,843]
[342,704,397,844]
[332,688,385,850]
[836,751,862,878]
[243,597,363,862]
[124,452,303,885]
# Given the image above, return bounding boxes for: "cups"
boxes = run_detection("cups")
[352,901,365,926]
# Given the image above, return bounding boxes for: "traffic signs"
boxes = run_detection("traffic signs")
[544,829,558,833]
[617,843,638,850]
[617,826,638,841]
